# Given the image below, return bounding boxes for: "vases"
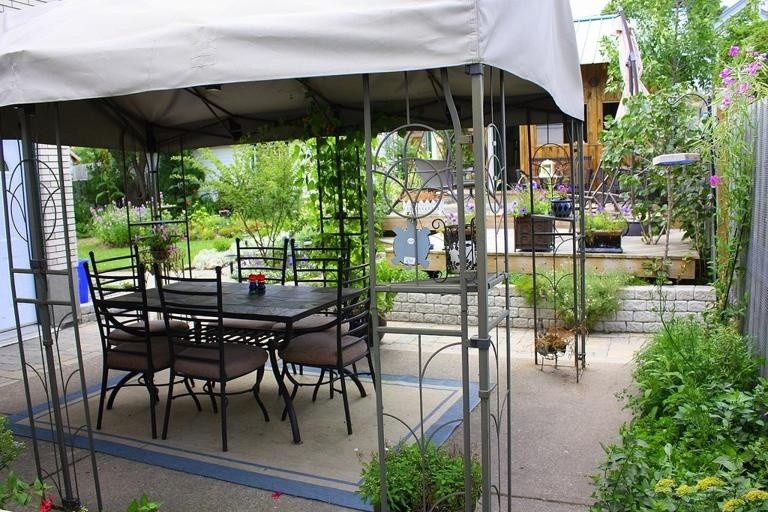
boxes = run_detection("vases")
[513,217,555,251]
[151,250,168,263]
[553,199,570,217]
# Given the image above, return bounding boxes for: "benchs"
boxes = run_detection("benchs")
[413,159,476,199]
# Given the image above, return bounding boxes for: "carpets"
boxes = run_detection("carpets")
[4,358,497,512]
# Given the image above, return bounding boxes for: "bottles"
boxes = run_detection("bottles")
[248,281,257,295]
[257,280,265,296]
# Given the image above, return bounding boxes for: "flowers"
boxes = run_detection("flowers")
[557,182,570,201]
[133,226,189,258]
[508,180,551,219]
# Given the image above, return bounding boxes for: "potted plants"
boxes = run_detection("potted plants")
[589,213,625,253]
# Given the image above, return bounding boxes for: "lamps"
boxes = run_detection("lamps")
[432,217,476,283]
